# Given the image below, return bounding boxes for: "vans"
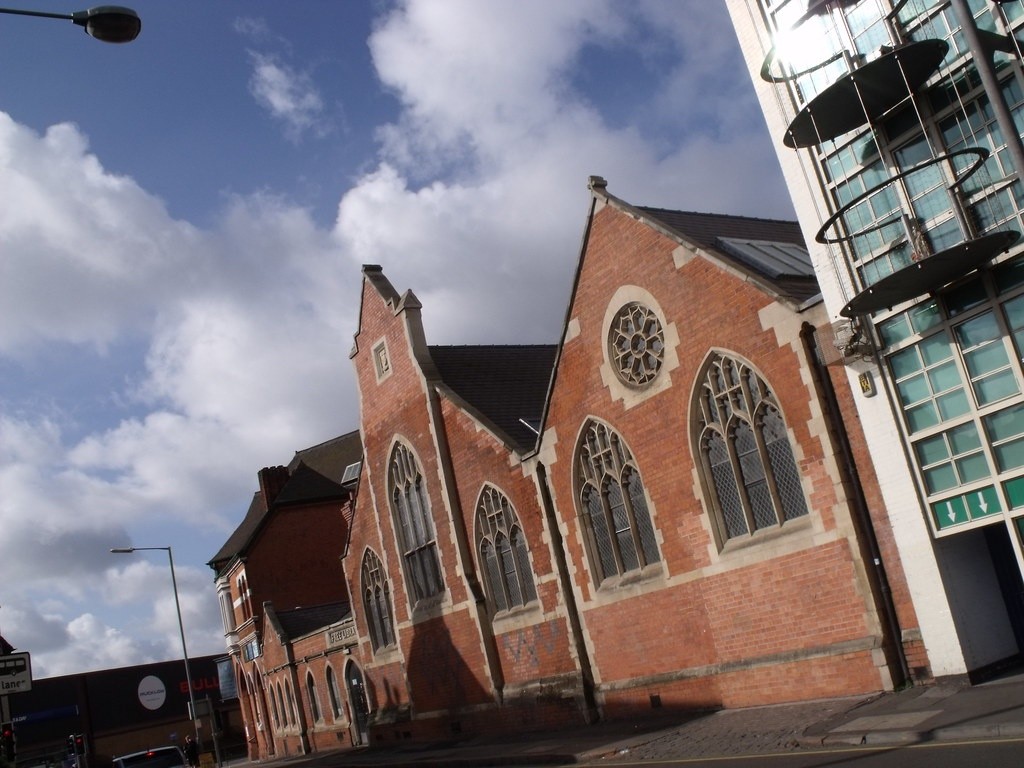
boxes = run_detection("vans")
[114,745,190,768]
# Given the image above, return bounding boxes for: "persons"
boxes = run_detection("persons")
[183,735,201,768]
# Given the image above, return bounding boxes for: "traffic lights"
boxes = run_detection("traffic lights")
[67,736,75,756]
[1,721,15,750]
[76,734,86,755]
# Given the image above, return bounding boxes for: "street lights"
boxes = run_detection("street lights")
[0,7,143,44]
[111,547,203,753]
